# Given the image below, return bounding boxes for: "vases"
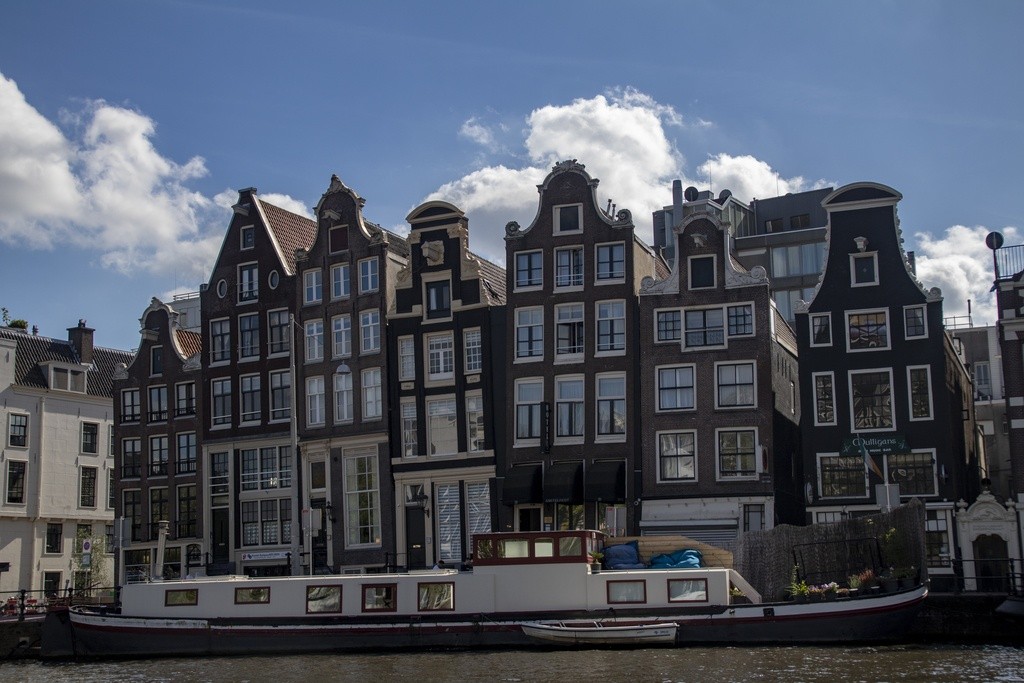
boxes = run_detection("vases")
[809,592,822,602]
[823,592,836,602]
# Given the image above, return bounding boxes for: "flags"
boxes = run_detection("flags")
[859,438,884,483]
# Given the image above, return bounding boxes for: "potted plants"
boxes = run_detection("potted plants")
[879,566,897,592]
[896,563,919,587]
[783,578,809,601]
[588,551,604,574]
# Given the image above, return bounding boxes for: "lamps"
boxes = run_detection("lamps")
[325,501,337,522]
[335,359,351,374]
[416,490,431,516]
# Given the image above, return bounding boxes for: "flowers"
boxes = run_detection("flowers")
[809,582,838,593]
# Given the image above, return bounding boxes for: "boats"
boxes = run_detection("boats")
[37,529,935,665]
[516,621,680,651]
